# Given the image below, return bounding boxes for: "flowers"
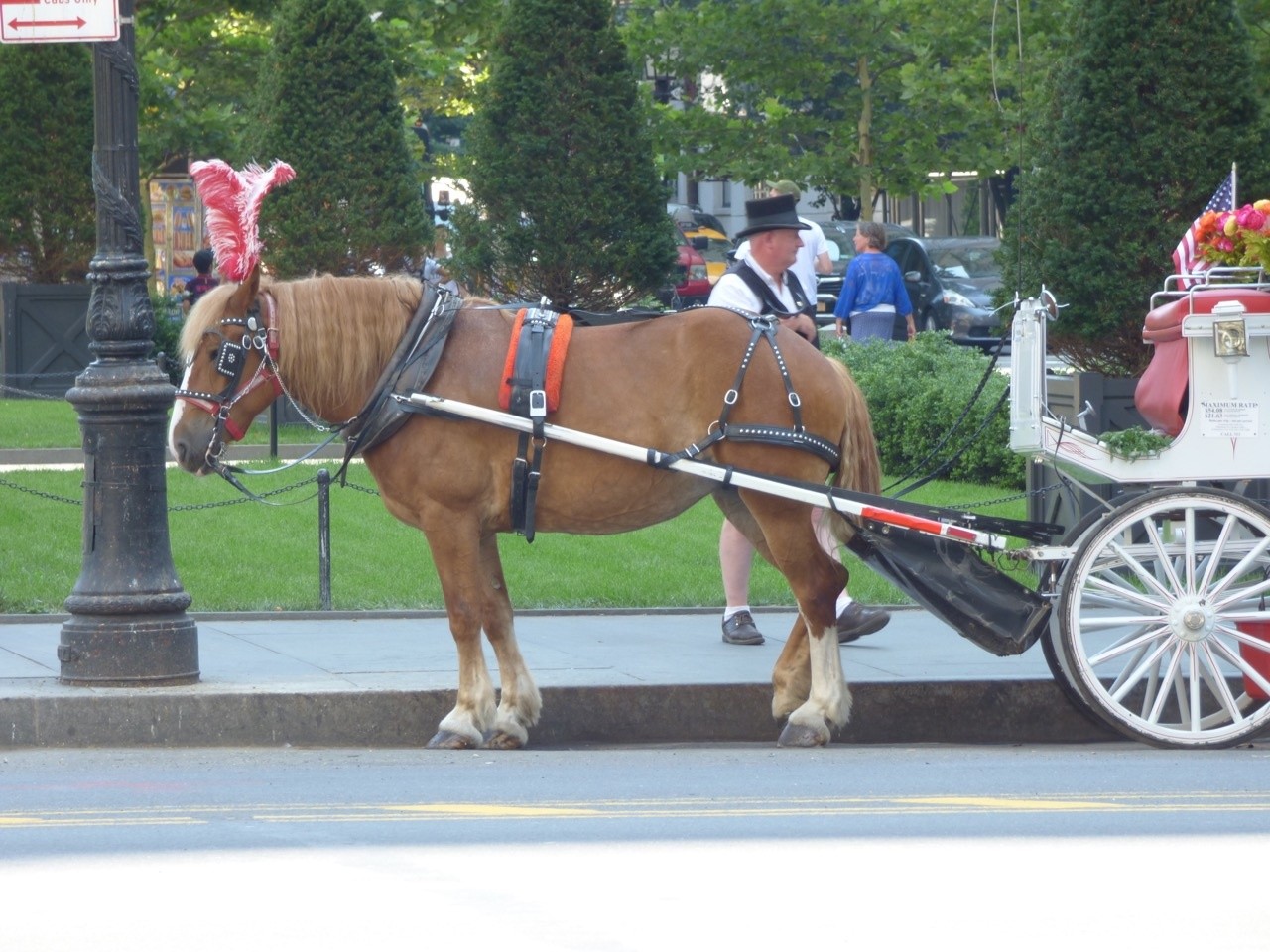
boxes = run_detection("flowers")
[1196,200,1270,276]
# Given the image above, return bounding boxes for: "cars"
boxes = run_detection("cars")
[874,238,1014,345]
[665,212,918,335]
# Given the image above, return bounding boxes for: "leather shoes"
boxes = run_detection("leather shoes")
[722,610,765,645]
[837,601,889,643]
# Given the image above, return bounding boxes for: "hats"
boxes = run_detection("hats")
[193,250,213,269]
[765,180,800,201]
[736,195,811,238]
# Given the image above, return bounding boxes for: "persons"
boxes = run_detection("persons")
[708,194,889,645]
[833,221,916,345]
[408,245,453,285]
[182,250,220,315]
[734,179,834,352]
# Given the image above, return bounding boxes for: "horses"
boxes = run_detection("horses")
[167,261,883,746]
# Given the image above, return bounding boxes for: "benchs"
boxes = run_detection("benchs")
[1136,265,1270,433]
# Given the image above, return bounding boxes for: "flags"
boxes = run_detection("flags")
[1172,168,1236,290]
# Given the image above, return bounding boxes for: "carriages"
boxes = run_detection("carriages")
[168,257,1269,754]
